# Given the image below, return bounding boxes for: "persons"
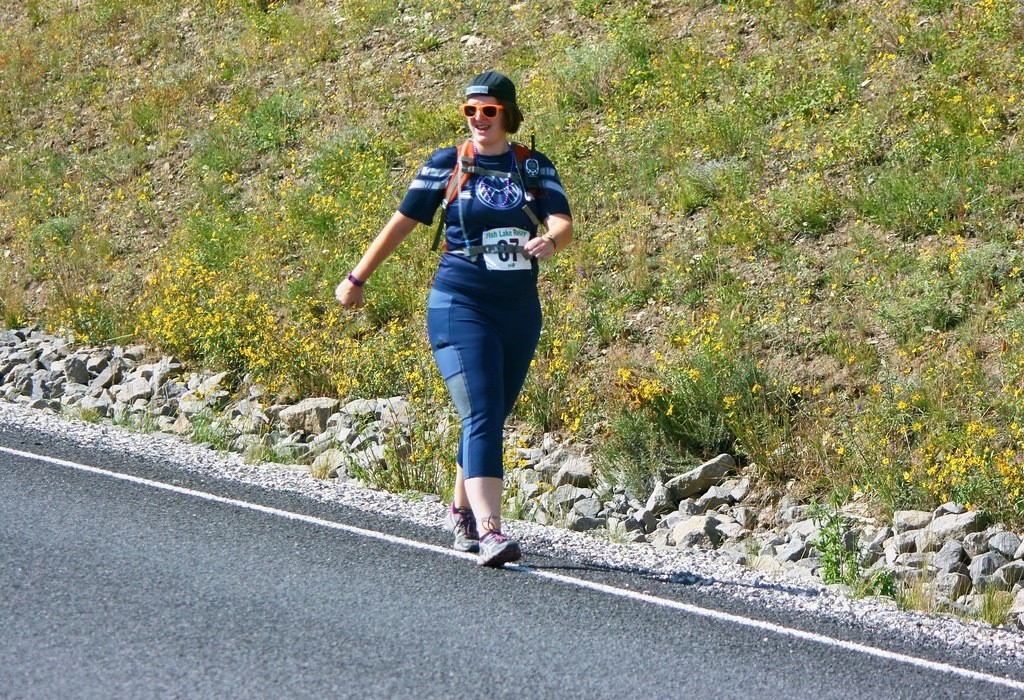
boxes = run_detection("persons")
[334,71,574,565]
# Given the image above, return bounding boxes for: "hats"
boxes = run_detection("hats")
[466,71,516,102]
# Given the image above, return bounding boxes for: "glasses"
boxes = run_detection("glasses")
[461,104,504,119]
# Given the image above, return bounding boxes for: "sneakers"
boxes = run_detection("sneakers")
[446,501,479,552]
[478,512,521,567]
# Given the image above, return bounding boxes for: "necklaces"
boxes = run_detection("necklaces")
[473,142,515,207]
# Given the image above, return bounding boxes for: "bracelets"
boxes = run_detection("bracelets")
[544,233,558,250]
[346,270,364,287]
